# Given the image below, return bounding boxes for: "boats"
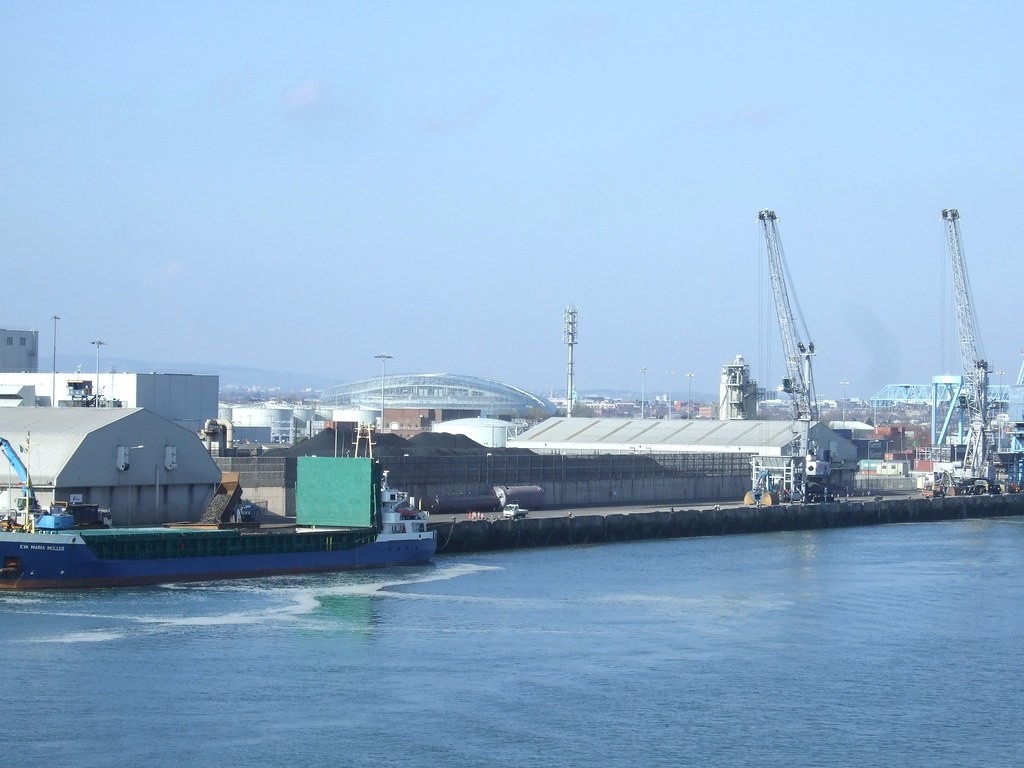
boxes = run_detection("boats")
[0,472,438,589]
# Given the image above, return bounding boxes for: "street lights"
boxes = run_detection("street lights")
[373,352,394,435]
[994,369,1005,454]
[839,377,850,427]
[666,369,676,420]
[51,315,62,407]
[685,373,694,419]
[639,366,648,420]
[90,337,107,409]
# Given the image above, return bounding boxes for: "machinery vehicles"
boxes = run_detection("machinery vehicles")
[0,437,74,531]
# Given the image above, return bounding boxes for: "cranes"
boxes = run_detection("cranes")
[758,208,820,420]
[940,205,996,473]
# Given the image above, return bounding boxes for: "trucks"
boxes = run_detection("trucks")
[50,502,113,529]
[503,503,529,519]
